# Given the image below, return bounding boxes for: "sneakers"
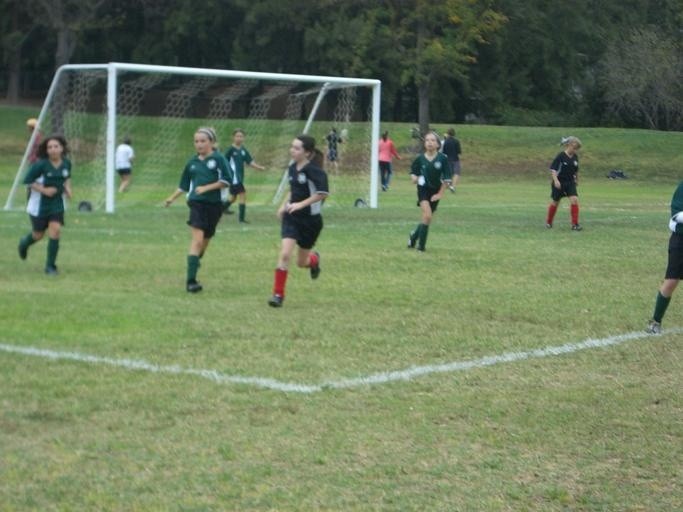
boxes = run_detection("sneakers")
[642,319,661,335]
[407,229,426,252]
[543,223,583,232]
[267,294,283,307]
[221,207,250,225]
[381,184,456,194]
[309,251,319,280]
[185,280,202,292]
[43,264,59,276]
[17,236,27,260]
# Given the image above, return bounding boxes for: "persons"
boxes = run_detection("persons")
[545,135,583,231]
[157,125,234,292]
[379,129,401,191]
[323,128,346,172]
[18,135,75,276]
[23,117,40,202]
[114,135,136,193]
[221,128,265,223]
[267,135,330,307]
[407,127,462,252]
[646,180,683,335]
